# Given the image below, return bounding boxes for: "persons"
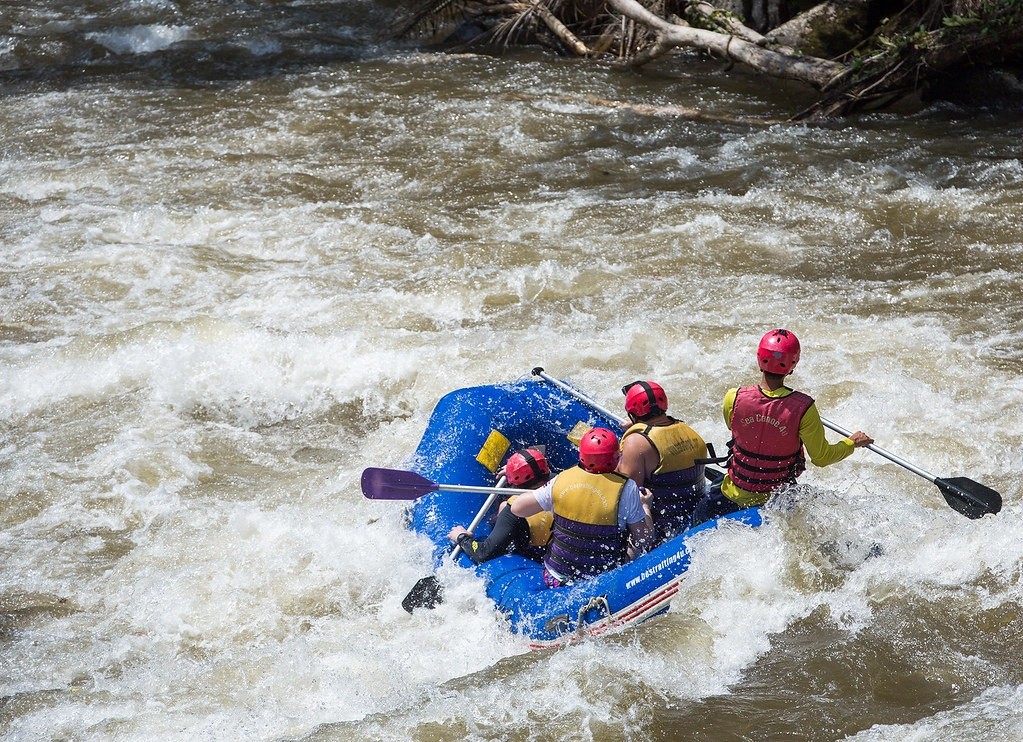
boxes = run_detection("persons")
[511,428,655,589]
[614,381,707,542]
[691,329,874,528]
[447,449,558,567]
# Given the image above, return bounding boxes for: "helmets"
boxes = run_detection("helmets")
[506,449,549,486]
[757,329,801,375]
[579,428,619,474]
[621,381,668,419]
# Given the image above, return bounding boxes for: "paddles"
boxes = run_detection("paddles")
[400,473,509,616]
[360,466,538,500]
[818,415,1003,521]
[530,364,728,484]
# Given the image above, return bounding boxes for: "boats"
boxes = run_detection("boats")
[402,381,768,650]
